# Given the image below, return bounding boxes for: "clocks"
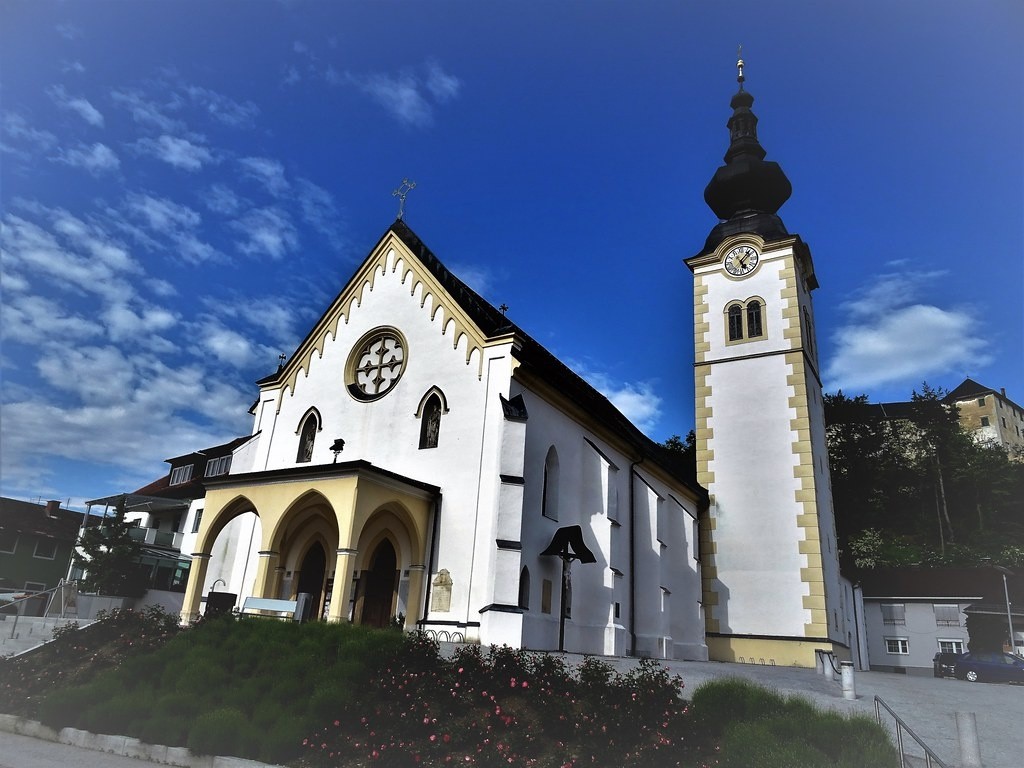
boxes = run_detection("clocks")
[724,246,759,276]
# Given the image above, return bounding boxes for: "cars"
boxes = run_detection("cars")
[932,652,964,679]
[954,650,1024,683]
